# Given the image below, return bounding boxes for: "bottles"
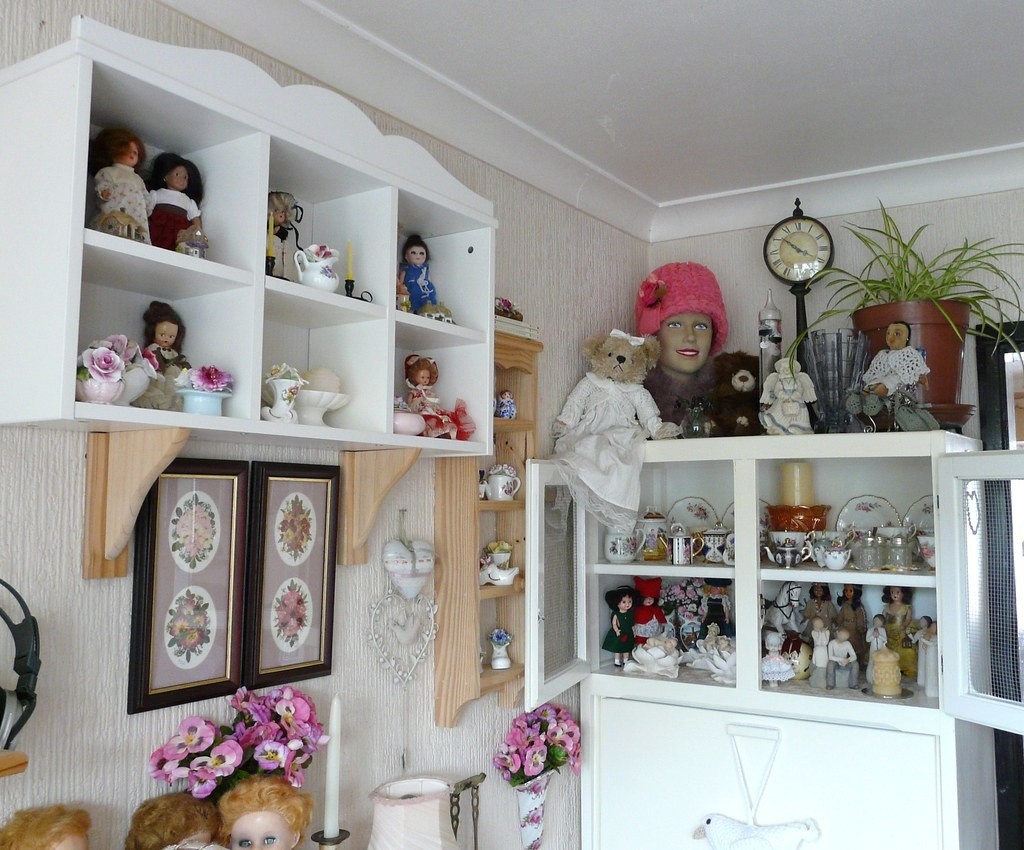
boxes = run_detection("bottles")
[856,534,913,570]
[638,511,669,560]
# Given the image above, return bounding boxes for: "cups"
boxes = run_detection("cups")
[487,552,511,569]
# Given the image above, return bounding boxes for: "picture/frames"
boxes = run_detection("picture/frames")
[244,459,341,692]
[126,456,249,714]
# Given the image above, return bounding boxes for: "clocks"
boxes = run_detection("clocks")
[764,197,836,424]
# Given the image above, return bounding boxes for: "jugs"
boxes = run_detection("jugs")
[604,522,646,564]
[656,519,735,566]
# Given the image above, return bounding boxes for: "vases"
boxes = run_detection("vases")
[76,376,123,404]
[479,552,520,586]
[491,643,511,670]
[116,368,153,406]
[368,777,449,850]
[295,391,348,426]
[515,767,560,850]
[174,388,231,417]
[485,476,520,501]
[292,250,340,292]
[604,522,646,564]
[392,411,426,436]
[261,379,299,423]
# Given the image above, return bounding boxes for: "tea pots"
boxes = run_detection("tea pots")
[484,474,521,501]
[763,534,852,570]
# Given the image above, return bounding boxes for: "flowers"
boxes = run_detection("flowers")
[489,463,517,477]
[393,397,411,410]
[494,296,518,316]
[480,540,514,571]
[488,628,512,646]
[303,244,339,263]
[172,364,233,393]
[495,702,582,787]
[263,363,309,387]
[76,334,166,384]
[147,684,330,799]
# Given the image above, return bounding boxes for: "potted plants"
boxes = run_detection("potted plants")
[781,199,1024,426]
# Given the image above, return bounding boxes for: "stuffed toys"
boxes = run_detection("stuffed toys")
[766,580,805,640]
[552,327,679,530]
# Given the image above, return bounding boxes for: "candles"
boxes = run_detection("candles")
[267,212,274,256]
[347,240,354,279]
[782,462,814,507]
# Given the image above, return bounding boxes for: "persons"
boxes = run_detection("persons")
[643,635,677,658]
[602,584,637,667]
[827,626,862,690]
[399,230,438,311]
[633,259,731,440]
[144,148,205,251]
[698,577,735,640]
[1,772,314,850]
[402,352,469,440]
[811,616,831,668]
[96,120,152,243]
[833,584,870,671]
[762,630,795,689]
[865,615,888,691]
[139,297,196,412]
[630,576,666,647]
[703,623,735,660]
[266,188,305,281]
[846,321,927,432]
[880,585,918,680]
[906,616,939,699]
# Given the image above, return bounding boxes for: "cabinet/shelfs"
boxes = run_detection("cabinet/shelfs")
[437,332,545,725]
[0,14,496,460]
[526,430,1024,850]
